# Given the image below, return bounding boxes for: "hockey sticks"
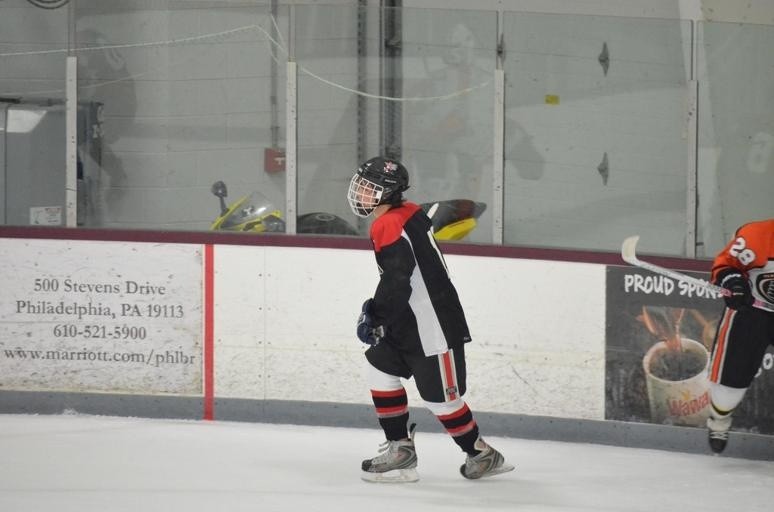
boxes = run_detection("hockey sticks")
[622,235,774,313]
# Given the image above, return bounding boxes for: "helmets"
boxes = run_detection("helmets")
[346,157,411,219]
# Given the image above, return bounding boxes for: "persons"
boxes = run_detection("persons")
[706,219,774,455]
[61,24,137,199]
[347,157,504,480]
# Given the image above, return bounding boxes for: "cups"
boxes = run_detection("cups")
[644,339,710,428]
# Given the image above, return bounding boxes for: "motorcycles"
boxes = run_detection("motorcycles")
[209,180,487,241]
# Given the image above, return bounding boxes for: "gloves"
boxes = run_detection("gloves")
[355,297,386,346]
[714,268,756,313]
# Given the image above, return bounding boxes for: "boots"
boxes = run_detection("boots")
[361,423,418,472]
[706,419,733,453]
[460,433,504,479]
